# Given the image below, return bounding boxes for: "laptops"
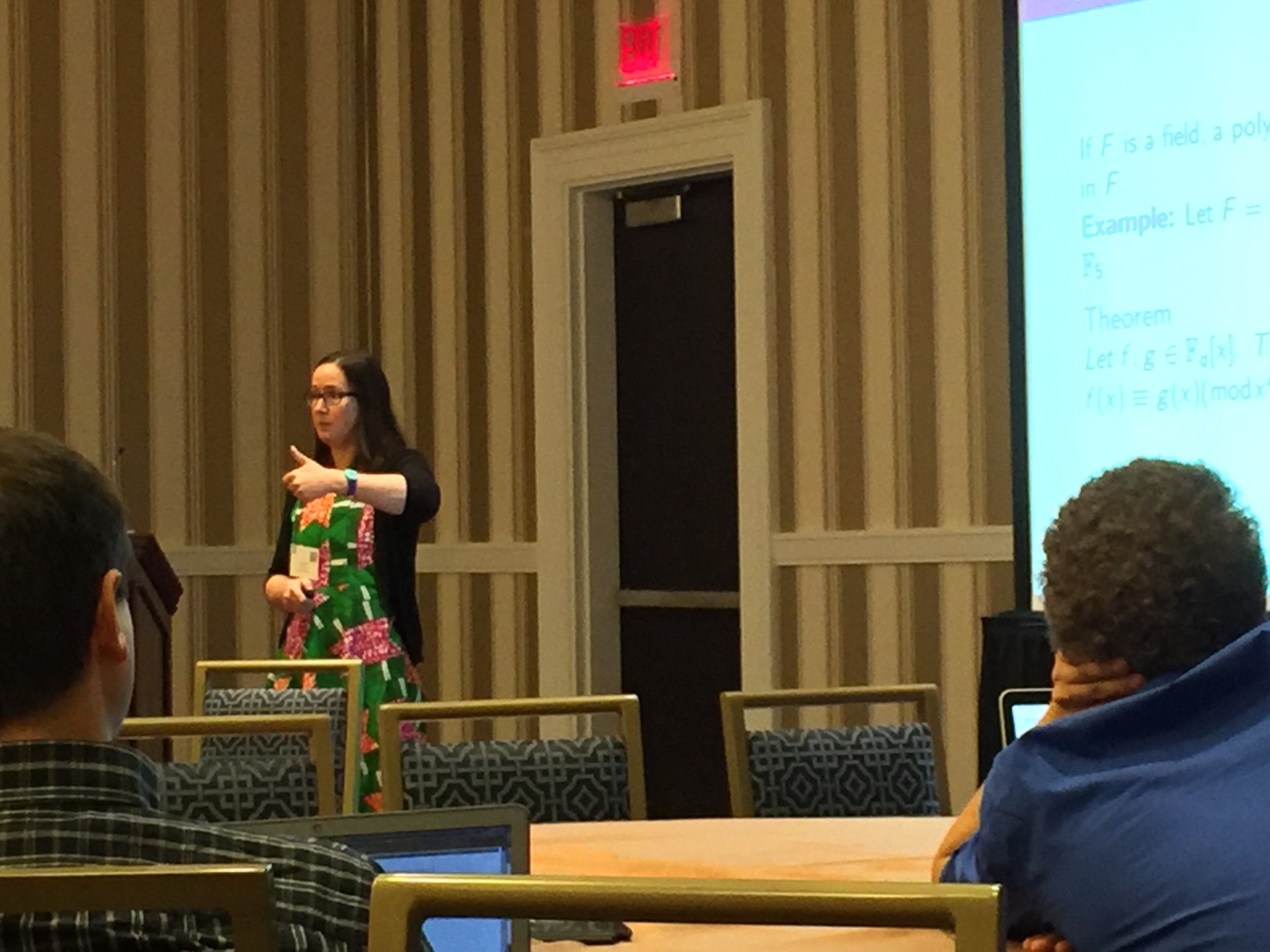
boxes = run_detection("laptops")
[999,687,1054,750]
[215,804,530,952]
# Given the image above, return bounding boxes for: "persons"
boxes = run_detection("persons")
[0,428,436,952]
[930,457,1269,952]
[264,350,440,816]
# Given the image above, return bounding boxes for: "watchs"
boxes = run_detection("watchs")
[342,467,358,496]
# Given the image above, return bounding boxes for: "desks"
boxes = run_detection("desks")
[526,814,967,952]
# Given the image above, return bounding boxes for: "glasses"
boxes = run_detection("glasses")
[304,390,357,406]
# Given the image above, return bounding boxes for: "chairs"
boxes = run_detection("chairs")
[1,660,1004,952]
